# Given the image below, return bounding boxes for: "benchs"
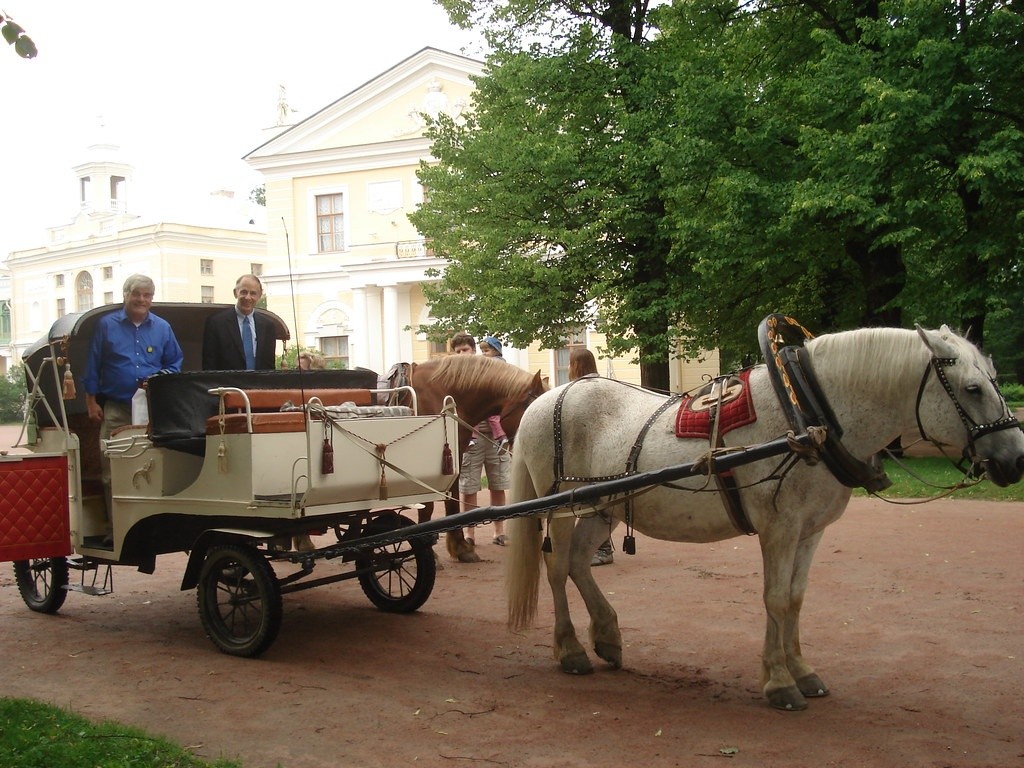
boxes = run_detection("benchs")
[207,385,418,435]
[110,368,378,440]
[52,411,106,498]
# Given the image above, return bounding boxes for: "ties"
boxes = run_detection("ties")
[242,315,255,370]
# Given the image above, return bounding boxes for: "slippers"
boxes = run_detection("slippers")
[466,538,475,547]
[493,534,511,546]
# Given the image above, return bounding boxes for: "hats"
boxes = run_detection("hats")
[481,337,502,355]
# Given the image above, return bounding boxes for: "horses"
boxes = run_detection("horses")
[502,322,1024,711]
[269,354,550,563]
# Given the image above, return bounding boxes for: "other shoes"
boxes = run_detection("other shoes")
[102,532,113,546]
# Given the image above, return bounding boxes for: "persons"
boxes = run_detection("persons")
[567,349,614,565]
[449,334,510,548]
[203,276,275,372]
[84,274,184,547]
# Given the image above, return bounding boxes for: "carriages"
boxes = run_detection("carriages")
[0,297,1024,711]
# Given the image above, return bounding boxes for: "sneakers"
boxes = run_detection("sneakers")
[590,549,614,566]
